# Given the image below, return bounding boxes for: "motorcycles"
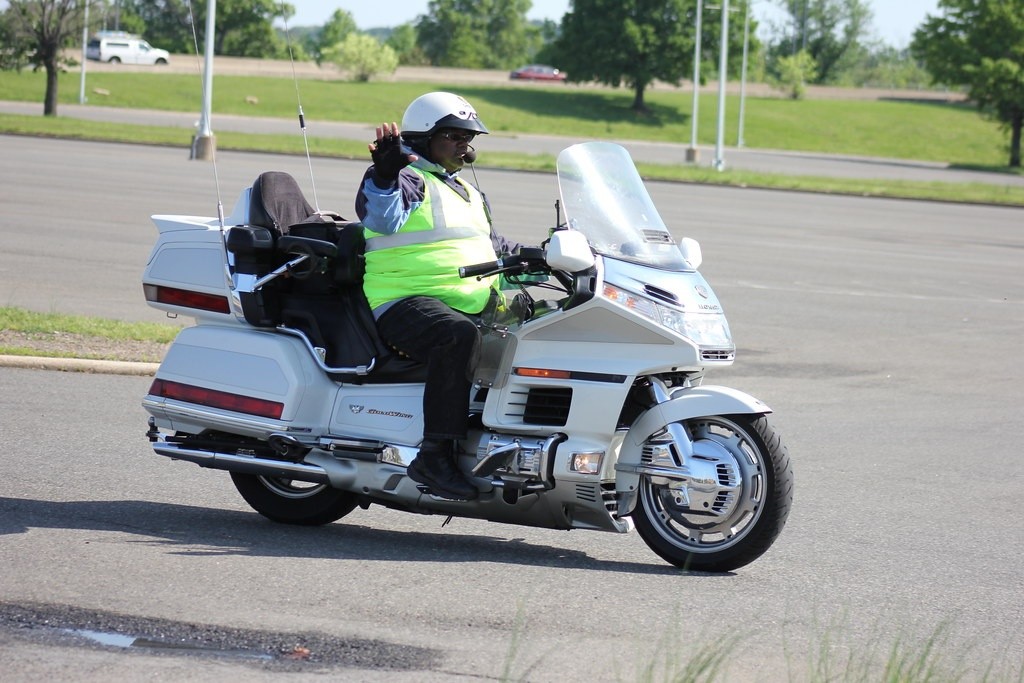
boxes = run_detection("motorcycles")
[140,144,793,574]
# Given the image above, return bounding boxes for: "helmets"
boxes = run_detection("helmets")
[400,91,489,137]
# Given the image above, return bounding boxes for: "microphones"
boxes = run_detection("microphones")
[463,144,477,163]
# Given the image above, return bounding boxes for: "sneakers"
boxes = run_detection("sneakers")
[407,436,479,500]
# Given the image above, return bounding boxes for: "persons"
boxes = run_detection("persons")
[354,91,555,499]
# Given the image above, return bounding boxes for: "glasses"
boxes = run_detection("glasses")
[436,133,474,143]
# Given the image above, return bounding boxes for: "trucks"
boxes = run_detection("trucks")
[87,33,171,65]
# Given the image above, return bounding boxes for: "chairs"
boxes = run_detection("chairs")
[231,171,431,384]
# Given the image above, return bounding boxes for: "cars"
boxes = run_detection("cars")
[510,65,568,80]
